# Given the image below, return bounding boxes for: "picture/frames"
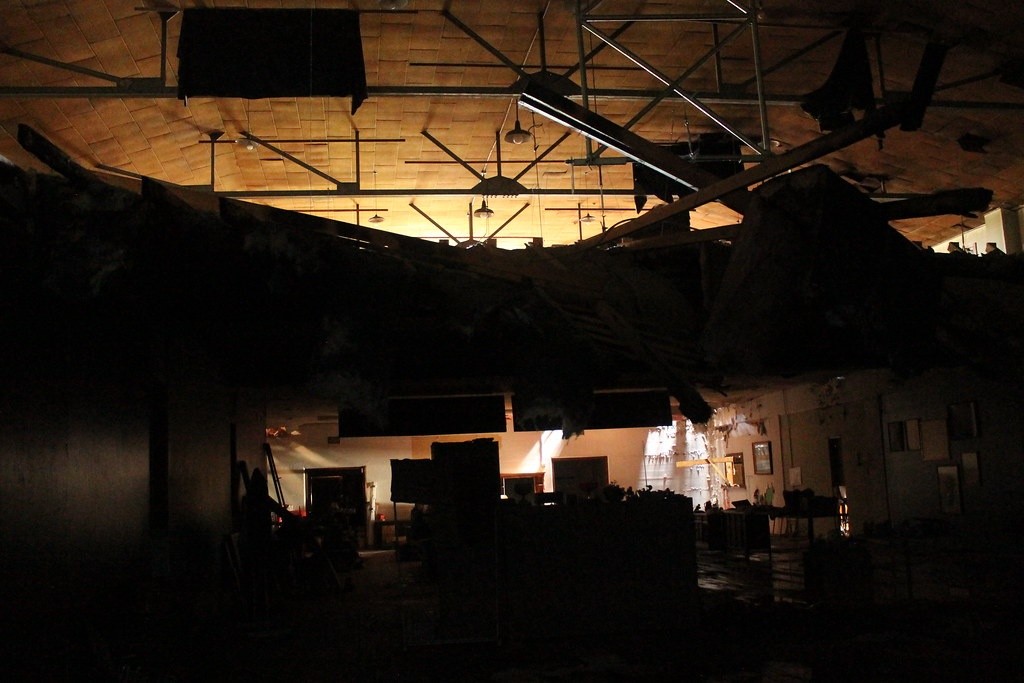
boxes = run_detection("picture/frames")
[752,441,773,476]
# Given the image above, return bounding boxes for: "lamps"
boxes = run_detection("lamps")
[234,135,259,150]
[369,170,383,222]
[578,172,596,222]
[505,97,531,143]
[475,169,493,219]
